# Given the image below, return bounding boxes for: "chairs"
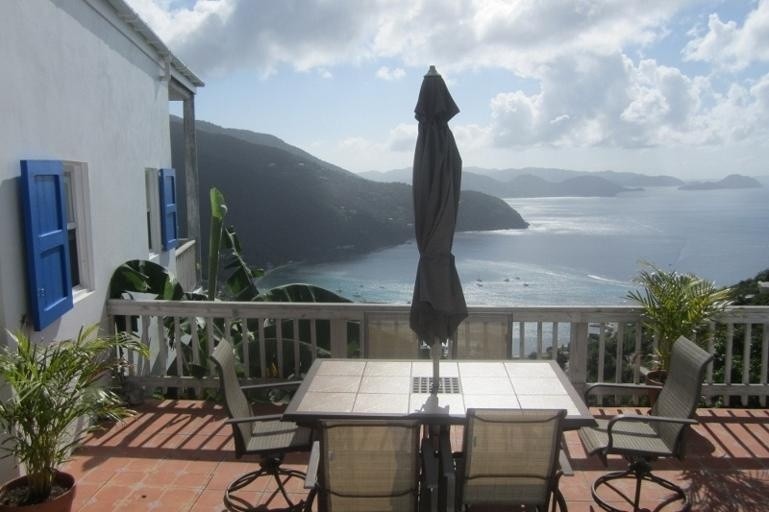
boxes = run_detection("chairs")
[302,415,437,512]
[577,334,715,511]
[209,335,314,511]
[433,404,570,512]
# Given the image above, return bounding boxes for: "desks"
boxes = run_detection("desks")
[280,355,599,450]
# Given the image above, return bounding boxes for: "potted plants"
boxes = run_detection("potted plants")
[623,256,739,409]
[0,320,149,512]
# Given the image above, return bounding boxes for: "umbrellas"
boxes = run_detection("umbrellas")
[410,64,469,388]
